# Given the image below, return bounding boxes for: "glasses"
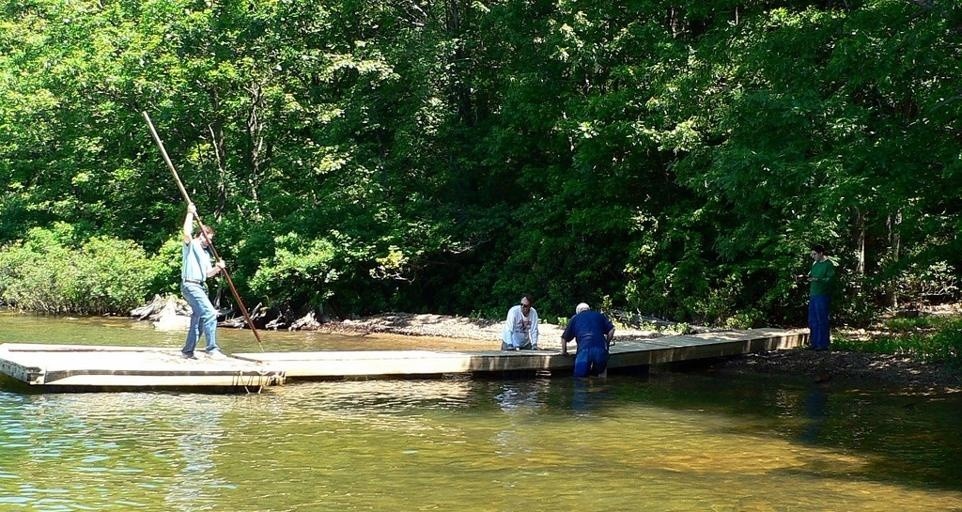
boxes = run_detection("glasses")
[519,302,532,308]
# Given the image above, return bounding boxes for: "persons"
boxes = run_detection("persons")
[790,244,836,351]
[560,302,616,379]
[180,201,227,362]
[501,292,540,351]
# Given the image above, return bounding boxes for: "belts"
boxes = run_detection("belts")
[183,279,205,287]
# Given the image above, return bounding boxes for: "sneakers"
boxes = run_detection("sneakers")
[203,347,228,363]
[178,347,199,363]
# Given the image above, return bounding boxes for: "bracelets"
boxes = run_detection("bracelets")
[188,210,194,214]
